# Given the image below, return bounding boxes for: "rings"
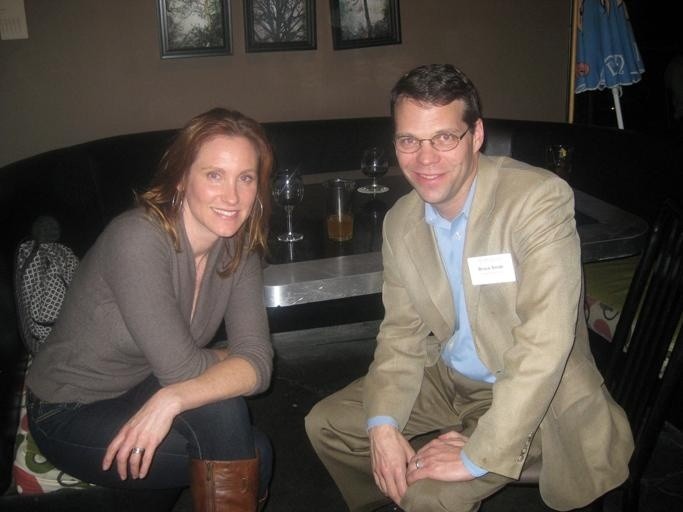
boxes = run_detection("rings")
[131,448,144,453]
[416,458,420,470]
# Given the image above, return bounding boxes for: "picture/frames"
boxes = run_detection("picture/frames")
[243,0,317,52]
[329,0,402,51]
[155,0,233,61]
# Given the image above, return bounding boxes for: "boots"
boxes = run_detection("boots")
[192,449,259,512]
[257,488,268,512]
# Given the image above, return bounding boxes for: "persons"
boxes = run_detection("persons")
[302,62,636,512]
[27,108,272,512]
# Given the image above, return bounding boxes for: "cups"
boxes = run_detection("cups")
[319,177,358,243]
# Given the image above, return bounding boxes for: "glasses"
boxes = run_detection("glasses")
[386,125,471,154]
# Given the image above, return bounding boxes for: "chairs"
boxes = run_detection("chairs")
[406,178,683,512]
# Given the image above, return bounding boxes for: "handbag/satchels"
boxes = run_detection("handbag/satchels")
[12,236,79,358]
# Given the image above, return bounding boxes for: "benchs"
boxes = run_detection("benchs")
[0,115,682,512]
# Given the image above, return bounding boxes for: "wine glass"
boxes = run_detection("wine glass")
[270,166,305,243]
[359,147,390,192]
